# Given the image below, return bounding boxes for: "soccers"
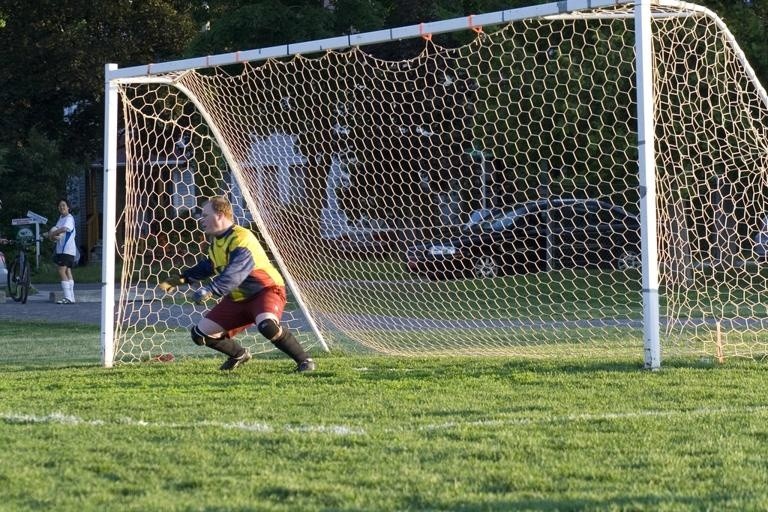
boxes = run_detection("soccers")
[50,226,65,241]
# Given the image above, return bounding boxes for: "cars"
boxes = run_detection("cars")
[404,197,668,281]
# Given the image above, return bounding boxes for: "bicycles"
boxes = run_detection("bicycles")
[5,236,42,303]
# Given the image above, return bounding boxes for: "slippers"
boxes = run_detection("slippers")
[56,298,74,304]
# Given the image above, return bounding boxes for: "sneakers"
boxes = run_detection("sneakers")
[298,358,316,371]
[219,347,252,371]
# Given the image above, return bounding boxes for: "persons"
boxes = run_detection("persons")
[158,195,319,373]
[47,198,77,305]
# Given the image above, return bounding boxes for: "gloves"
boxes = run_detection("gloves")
[190,287,214,305]
[164,273,181,287]
